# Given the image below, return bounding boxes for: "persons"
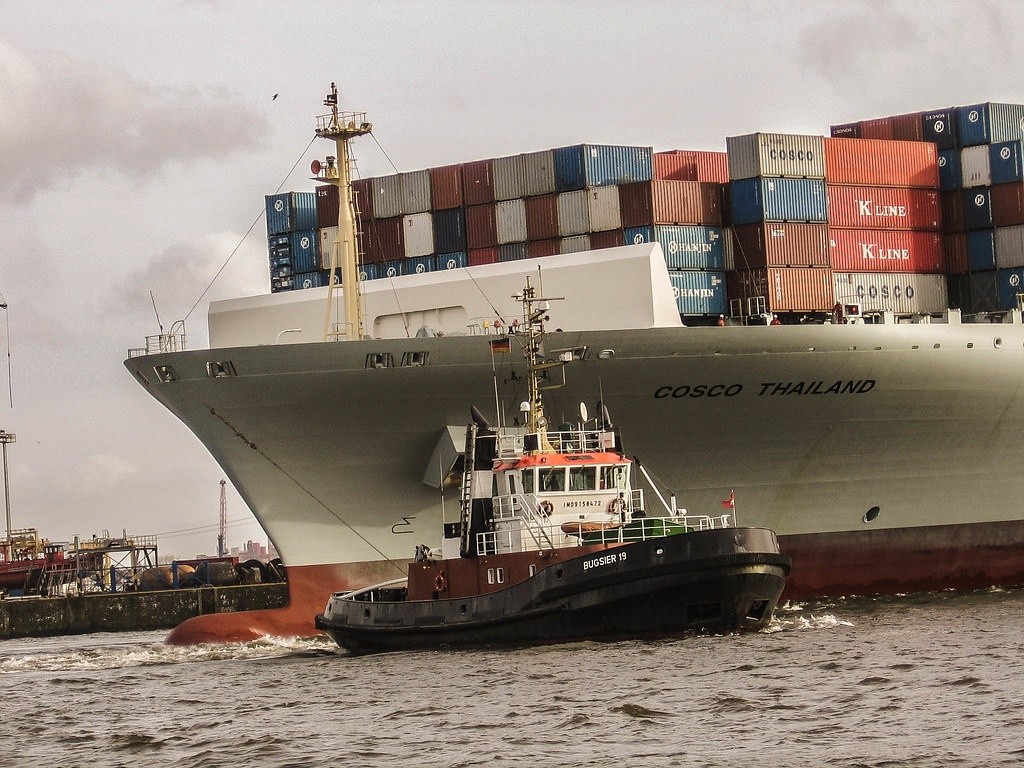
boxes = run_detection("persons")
[718,314,725,327]
[833,299,844,324]
[546,474,560,491]
[770,314,780,325]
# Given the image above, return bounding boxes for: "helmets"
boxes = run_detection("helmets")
[774,315,777,317]
[720,314,724,317]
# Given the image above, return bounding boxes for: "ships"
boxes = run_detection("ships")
[122,79,1023,650]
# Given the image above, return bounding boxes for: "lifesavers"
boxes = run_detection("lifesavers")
[239,559,266,582]
[612,498,625,514]
[266,558,285,582]
[434,575,445,591]
[540,500,553,518]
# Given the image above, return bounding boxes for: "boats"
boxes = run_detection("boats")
[316,273,792,642]
[0,427,105,595]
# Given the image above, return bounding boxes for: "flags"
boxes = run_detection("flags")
[722,491,735,508]
